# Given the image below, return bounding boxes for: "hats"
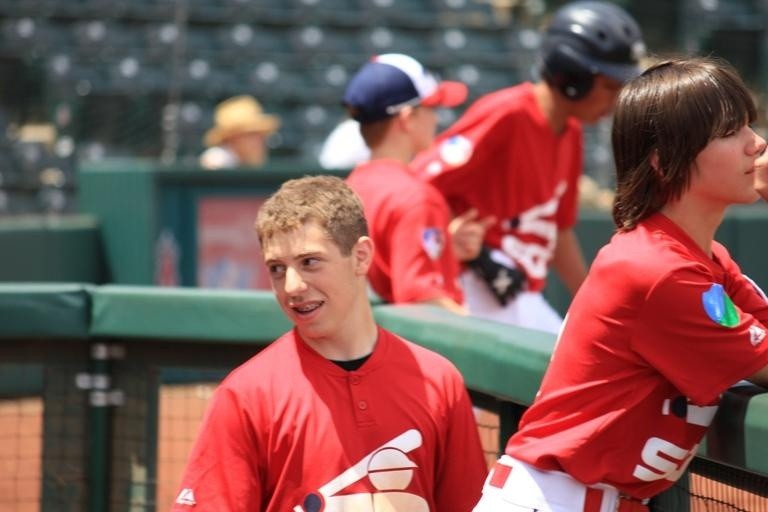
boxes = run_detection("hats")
[202,96,283,147]
[341,54,468,121]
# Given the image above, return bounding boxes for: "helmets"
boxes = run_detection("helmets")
[540,2,648,99]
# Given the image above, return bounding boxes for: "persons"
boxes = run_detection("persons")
[174,174,488,511]
[339,46,498,319]
[198,98,280,170]
[411,0,646,338]
[477,55,768,512]
